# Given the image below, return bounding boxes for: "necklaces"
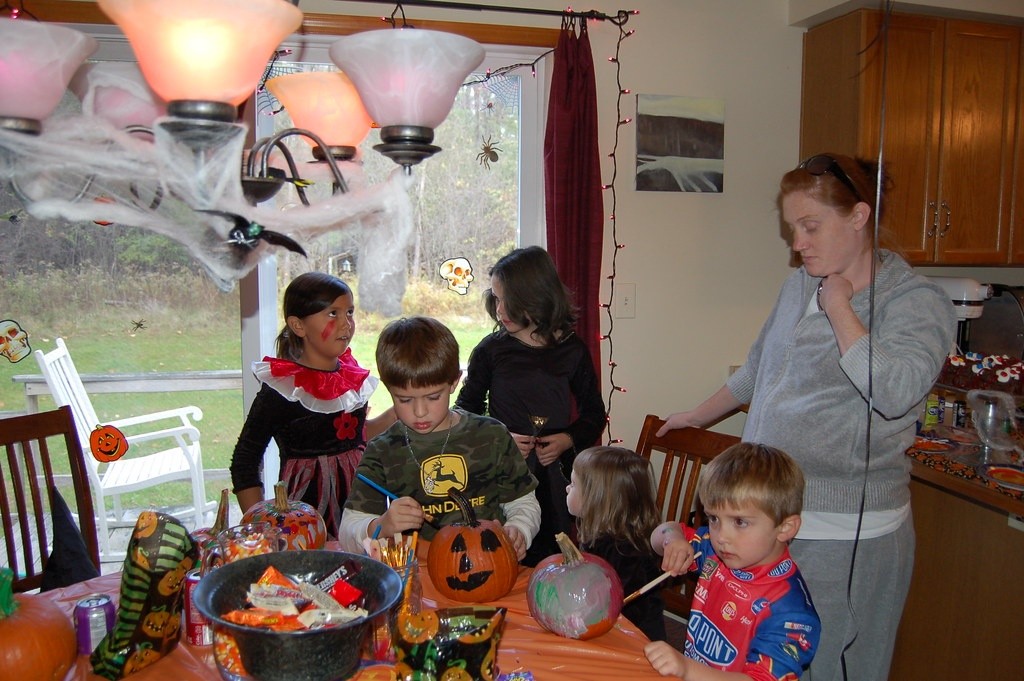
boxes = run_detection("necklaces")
[405,413,453,495]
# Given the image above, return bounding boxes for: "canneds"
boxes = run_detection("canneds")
[952,400,966,428]
[1000,415,1011,435]
[73,593,115,657]
[185,568,215,647]
[938,396,945,423]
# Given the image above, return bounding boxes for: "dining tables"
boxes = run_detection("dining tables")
[36,539,683,681]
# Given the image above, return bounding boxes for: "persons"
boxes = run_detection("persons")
[338,317,541,561]
[456,246,606,569]
[656,153,954,681]
[645,443,823,681]
[229,272,398,537]
[566,446,666,641]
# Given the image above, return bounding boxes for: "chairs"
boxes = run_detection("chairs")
[636,414,742,530]
[34,338,218,561]
[0,404,100,593]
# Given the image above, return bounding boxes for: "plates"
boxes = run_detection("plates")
[975,462,1024,492]
[910,436,961,455]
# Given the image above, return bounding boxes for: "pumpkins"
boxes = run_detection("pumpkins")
[185,489,245,570]
[426,486,518,602]
[0,567,77,681]
[238,480,327,553]
[527,532,624,640]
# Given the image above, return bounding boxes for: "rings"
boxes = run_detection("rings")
[817,287,823,294]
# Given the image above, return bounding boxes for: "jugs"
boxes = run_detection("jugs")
[199,521,284,681]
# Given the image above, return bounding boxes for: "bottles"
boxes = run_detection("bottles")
[369,557,423,664]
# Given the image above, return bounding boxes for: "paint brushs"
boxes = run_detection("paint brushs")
[623,551,703,607]
[356,473,441,531]
[362,524,419,611]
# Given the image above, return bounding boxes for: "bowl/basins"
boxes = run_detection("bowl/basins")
[191,550,404,681]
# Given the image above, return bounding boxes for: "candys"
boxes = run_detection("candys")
[225,533,270,562]
[213,566,369,676]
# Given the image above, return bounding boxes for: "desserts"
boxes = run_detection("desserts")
[938,349,1024,397]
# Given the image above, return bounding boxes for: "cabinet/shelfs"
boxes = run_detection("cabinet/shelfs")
[798,7,1024,269]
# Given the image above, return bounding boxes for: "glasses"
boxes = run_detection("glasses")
[799,156,864,204]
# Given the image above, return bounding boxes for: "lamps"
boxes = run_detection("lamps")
[0,0,486,296]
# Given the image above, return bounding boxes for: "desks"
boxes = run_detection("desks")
[13,370,242,486]
